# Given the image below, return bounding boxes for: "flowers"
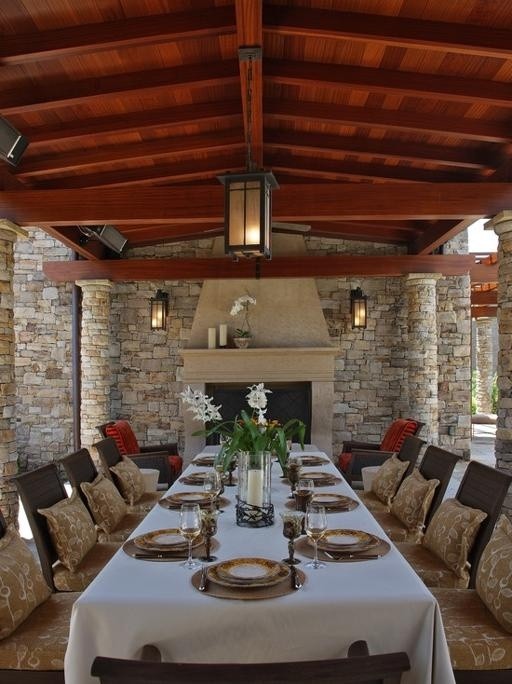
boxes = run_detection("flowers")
[180,383,306,478]
[230,293,257,336]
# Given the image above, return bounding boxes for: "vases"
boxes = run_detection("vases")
[234,450,275,526]
[236,338,251,348]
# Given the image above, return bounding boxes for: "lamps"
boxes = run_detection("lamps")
[350,287,368,328]
[149,289,168,329]
[216,142,281,262]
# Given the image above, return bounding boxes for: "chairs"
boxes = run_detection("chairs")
[59,449,148,540]
[11,464,125,592]
[0,506,86,684]
[393,462,510,589]
[91,421,182,511]
[368,444,464,540]
[335,418,427,513]
[91,639,411,684]
[430,590,512,684]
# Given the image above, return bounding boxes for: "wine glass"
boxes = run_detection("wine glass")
[304,504,328,568]
[204,470,221,515]
[279,510,305,564]
[179,503,201,569]
[198,510,218,562]
[286,464,301,498]
[297,479,314,519]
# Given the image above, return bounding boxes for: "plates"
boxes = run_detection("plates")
[134,528,204,551]
[165,491,211,505]
[216,557,282,583]
[188,470,215,482]
[196,456,217,463]
[309,493,354,507]
[292,456,323,463]
[307,528,381,552]
[205,563,290,589]
[302,472,335,482]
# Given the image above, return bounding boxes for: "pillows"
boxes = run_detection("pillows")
[390,467,440,543]
[36,487,98,575]
[79,473,126,533]
[475,514,512,635]
[423,499,489,579]
[1,524,51,642]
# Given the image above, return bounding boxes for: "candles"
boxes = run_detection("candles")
[248,469,263,523]
[208,323,227,348]
